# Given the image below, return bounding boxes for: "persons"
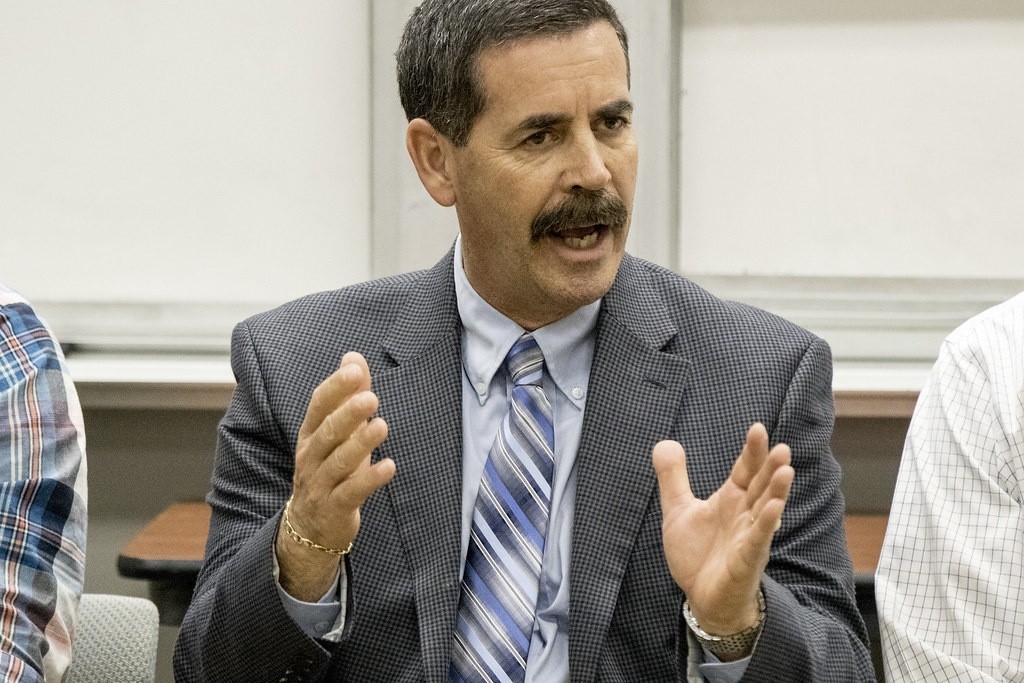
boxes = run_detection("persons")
[172,0,876,682]
[875,292,1024,683]
[0,289,88,682]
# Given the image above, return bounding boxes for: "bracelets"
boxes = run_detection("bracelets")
[683,589,766,654]
[284,499,352,554]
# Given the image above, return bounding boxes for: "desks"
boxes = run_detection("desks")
[118,502,889,609]
